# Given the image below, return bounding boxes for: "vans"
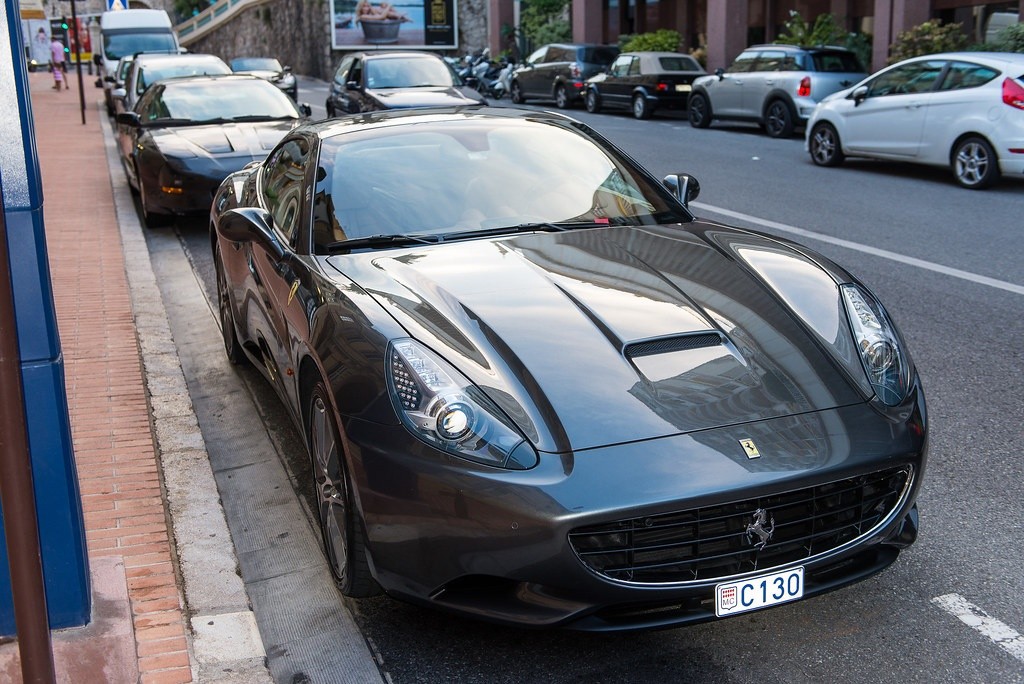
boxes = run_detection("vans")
[93,8,189,106]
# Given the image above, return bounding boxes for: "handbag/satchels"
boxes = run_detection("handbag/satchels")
[53,66,62,82]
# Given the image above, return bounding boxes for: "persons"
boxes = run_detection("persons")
[354,0,415,27]
[49,36,69,89]
[49,60,63,92]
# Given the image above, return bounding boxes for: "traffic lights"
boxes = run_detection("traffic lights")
[63,40,69,53]
[61,17,68,30]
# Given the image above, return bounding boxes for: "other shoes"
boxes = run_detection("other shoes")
[52,86,61,89]
[66,87,68,89]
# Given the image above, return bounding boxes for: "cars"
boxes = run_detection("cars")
[579,49,708,121]
[115,72,315,231]
[231,119,930,638]
[229,57,299,105]
[111,52,234,113]
[104,55,134,90]
[804,51,1024,191]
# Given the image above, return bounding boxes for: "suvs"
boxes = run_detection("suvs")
[684,41,870,140]
[325,47,491,121]
[509,43,621,109]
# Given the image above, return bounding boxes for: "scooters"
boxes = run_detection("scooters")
[447,49,506,100]
